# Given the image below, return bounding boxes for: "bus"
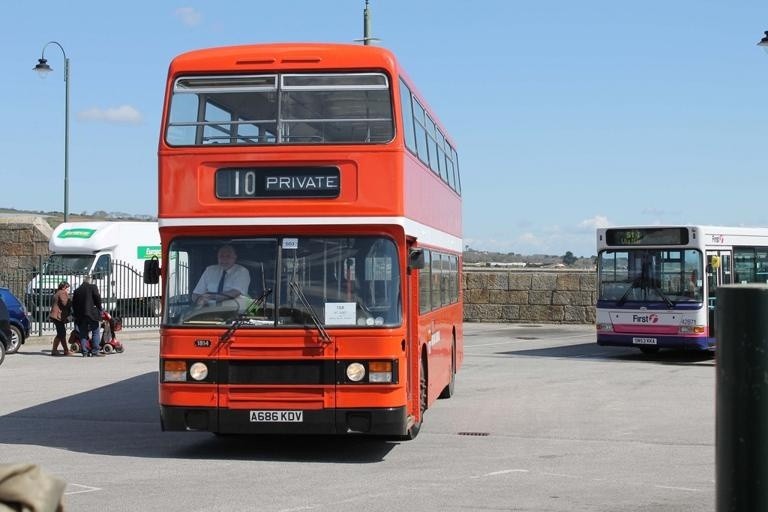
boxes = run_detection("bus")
[156,42,466,443]
[593,223,768,358]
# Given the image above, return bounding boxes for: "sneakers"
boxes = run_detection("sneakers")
[51,348,108,358]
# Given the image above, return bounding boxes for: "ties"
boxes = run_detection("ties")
[216,269,227,307]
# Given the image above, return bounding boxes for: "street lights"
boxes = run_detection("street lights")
[32,41,71,223]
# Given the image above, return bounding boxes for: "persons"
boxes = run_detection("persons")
[73,282,105,357]
[191,244,252,315]
[50,282,73,355]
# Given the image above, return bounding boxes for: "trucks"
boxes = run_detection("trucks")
[24,221,162,320]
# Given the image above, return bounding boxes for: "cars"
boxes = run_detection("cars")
[0,286,31,366]
